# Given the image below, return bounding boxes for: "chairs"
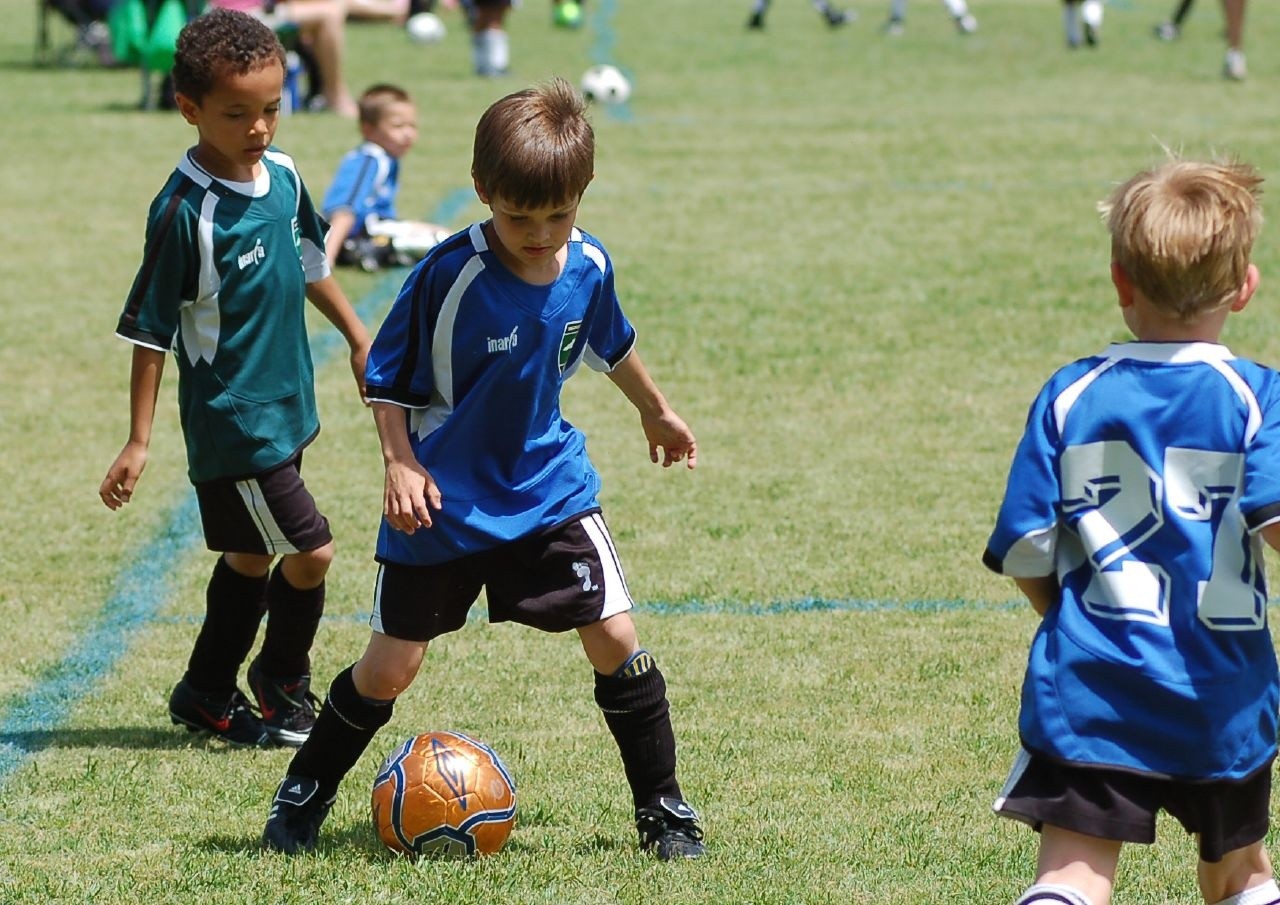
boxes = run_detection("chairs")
[34,0,322,118]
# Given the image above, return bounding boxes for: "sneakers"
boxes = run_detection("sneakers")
[168,680,268,748]
[635,797,704,862]
[248,654,324,746]
[264,778,337,856]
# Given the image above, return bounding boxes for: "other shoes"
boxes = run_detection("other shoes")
[1083,15,1102,47]
[959,17,976,35]
[1155,21,1178,44]
[1225,52,1248,81]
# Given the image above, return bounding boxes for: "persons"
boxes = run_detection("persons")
[881,0,976,36]
[35,0,511,116]
[1222,0,1251,84]
[317,82,455,274]
[96,5,371,748]
[982,137,1280,904]
[1062,0,1098,50]
[263,77,709,866]
[748,0,849,30]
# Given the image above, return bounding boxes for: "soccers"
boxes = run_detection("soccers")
[404,12,447,44]
[580,63,632,108]
[370,729,517,860]
